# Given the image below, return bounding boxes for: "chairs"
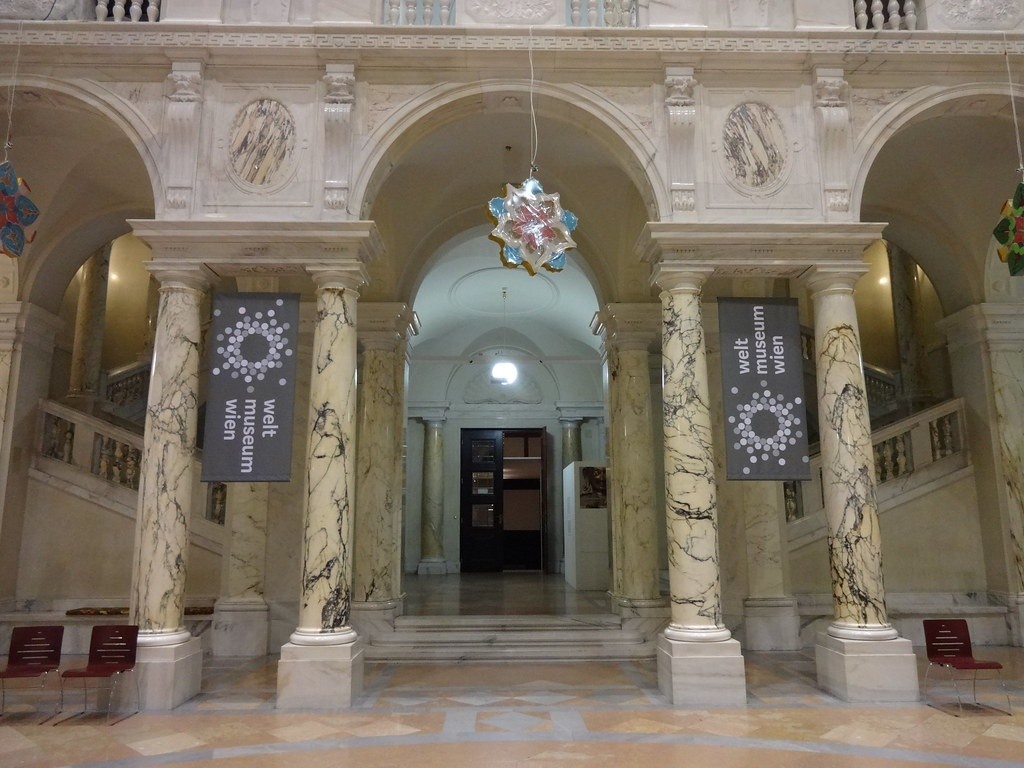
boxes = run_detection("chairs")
[53,625,139,727]
[0,626,64,716]
[922,619,1014,717]
